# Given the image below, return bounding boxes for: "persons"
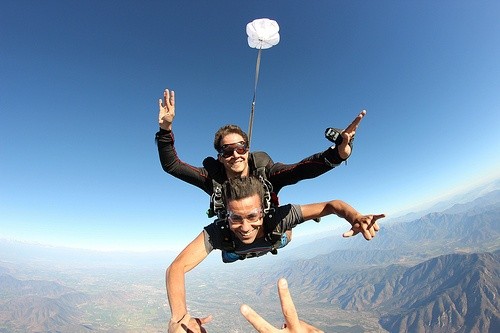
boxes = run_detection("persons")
[235,277,331,333]
[152,88,368,206]
[157,177,389,333]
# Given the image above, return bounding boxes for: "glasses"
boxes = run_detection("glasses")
[219,141,249,158]
[226,211,264,223]
